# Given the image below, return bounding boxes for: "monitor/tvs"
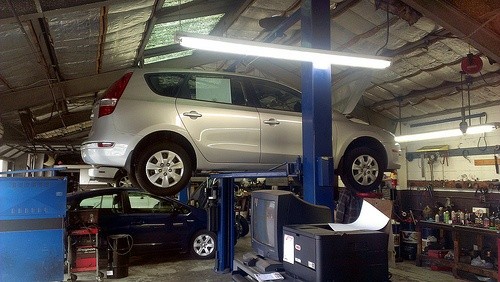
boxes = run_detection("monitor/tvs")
[250,190,332,263]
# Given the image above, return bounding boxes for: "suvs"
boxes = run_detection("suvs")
[78,66,404,197]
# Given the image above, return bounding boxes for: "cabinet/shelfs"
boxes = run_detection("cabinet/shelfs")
[68,208,104,281]
[400,187,500,252]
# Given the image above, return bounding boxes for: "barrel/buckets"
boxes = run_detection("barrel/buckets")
[391,221,402,262]
[400,221,421,261]
[422,221,440,254]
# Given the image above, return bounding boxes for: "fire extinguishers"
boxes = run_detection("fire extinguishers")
[390,169,398,190]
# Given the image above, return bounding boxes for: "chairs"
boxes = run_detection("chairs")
[112,196,120,214]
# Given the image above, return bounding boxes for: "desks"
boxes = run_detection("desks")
[417,210,499,281]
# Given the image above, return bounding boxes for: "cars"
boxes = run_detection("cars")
[67,188,237,260]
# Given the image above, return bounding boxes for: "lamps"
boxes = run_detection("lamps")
[175,0,393,77]
[393,86,499,144]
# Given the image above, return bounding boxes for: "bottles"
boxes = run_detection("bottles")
[435,214,439,223]
[451,208,471,225]
[490,212,500,229]
[483,217,490,227]
[445,211,450,224]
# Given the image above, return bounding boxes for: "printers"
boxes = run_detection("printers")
[282,223,388,282]
